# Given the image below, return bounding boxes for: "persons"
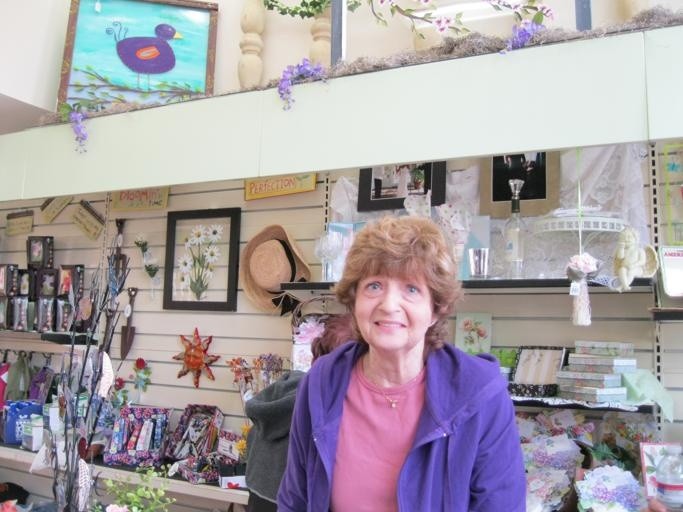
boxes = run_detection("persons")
[277,216,526,512]
[245,314,365,512]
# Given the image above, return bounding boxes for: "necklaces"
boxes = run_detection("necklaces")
[362,366,425,411]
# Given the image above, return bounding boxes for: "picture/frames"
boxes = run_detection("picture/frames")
[357,157,446,212]
[330,0,594,64]
[479,144,561,219]
[57,0,219,118]
[162,208,241,312]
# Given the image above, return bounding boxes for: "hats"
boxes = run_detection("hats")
[240,224,311,314]
